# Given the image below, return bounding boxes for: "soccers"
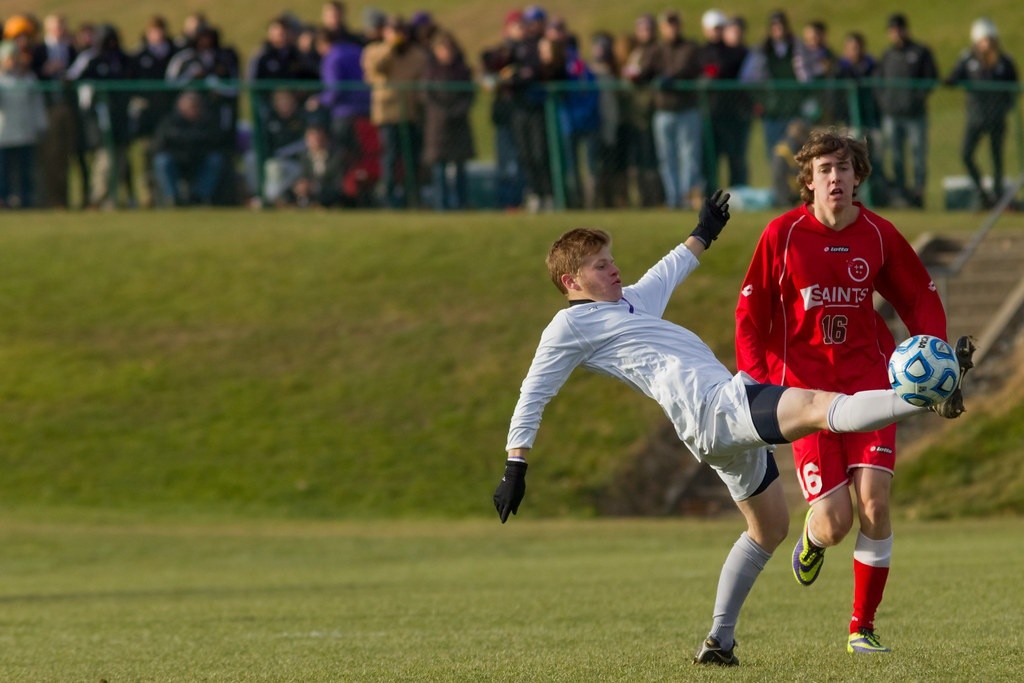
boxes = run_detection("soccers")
[887,334,959,405]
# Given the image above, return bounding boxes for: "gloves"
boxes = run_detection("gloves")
[493,457,529,524]
[686,190,731,250]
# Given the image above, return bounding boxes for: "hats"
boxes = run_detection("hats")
[502,11,523,29]
[637,14,653,31]
[886,14,906,29]
[700,10,727,30]
[3,15,32,39]
[524,8,546,21]
[545,17,565,33]
[412,10,431,27]
[970,18,1000,46]
[366,11,386,29]
[767,10,788,25]
[387,15,408,32]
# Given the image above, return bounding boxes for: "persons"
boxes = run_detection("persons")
[493,189,976,665]
[735,130,950,651]
[1,0,1023,211]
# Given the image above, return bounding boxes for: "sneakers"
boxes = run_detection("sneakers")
[792,507,825,586]
[847,626,891,654]
[691,635,739,667]
[929,336,975,419]
